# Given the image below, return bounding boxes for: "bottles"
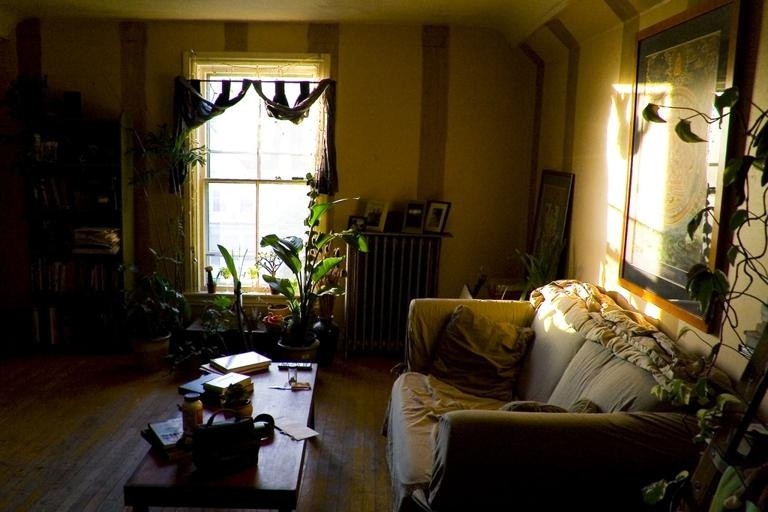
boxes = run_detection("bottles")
[181,393,203,433]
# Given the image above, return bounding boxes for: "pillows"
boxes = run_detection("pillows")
[426,305,536,401]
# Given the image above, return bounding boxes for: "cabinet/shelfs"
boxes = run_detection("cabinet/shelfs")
[18,111,135,357]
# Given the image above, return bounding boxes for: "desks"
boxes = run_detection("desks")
[185,317,312,359]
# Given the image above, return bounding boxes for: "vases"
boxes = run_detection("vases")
[312,314,339,368]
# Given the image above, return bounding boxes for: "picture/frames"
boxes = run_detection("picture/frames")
[524,168,574,282]
[363,200,389,232]
[401,200,424,233]
[618,0,751,333]
[347,215,367,232]
[423,200,451,233]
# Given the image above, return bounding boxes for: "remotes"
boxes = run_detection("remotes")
[278,362,312,371]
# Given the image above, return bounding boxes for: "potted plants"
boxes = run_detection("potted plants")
[281,298,301,333]
[203,264,230,293]
[238,304,262,330]
[200,296,243,329]
[260,172,369,363]
[216,243,247,325]
[115,263,191,370]
[256,295,289,317]
[253,249,284,295]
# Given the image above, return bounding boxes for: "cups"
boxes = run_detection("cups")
[288,368,297,384]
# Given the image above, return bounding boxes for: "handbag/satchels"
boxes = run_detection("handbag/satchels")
[194,410,273,477]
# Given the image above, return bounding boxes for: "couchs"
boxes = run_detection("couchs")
[381,278,767,512]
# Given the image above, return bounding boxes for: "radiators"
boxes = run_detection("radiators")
[342,231,453,364]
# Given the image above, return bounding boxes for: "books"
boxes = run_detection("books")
[141,416,183,463]
[32,173,86,208]
[34,133,61,164]
[204,371,254,405]
[34,258,121,295]
[180,372,224,396]
[199,351,272,374]
[33,307,115,345]
[72,226,121,257]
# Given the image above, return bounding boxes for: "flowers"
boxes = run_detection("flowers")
[312,228,347,318]
